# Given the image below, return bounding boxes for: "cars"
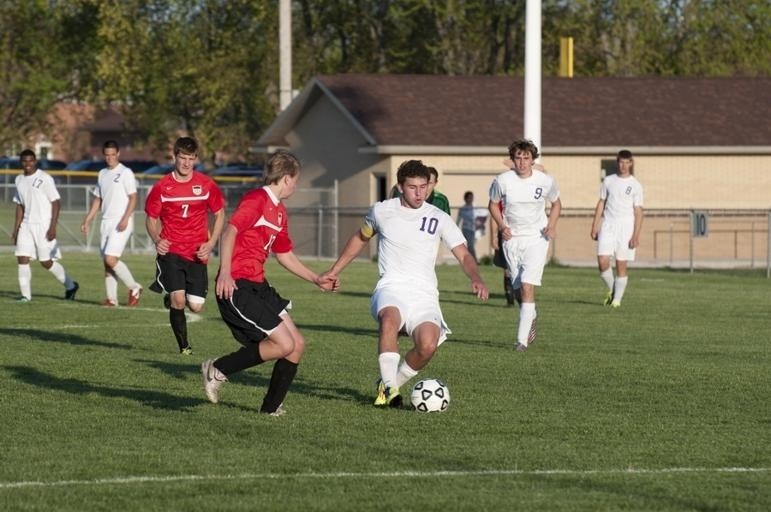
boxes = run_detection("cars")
[0,159,265,206]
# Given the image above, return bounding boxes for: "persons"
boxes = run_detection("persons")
[145,138,227,354]
[12,149,78,302]
[318,160,489,408]
[487,140,562,350]
[426,166,451,214]
[199,150,340,417]
[456,191,477,262]
[592,150,644,311]
[80,141,142,308]
[495,200,523,308]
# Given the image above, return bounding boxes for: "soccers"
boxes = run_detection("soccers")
[410,378,451,412]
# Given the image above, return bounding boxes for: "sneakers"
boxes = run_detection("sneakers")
[66,282,79,300]
[103,300,119,307]
[202,358,229,403]
[604,292,614,306]
[374,379,387,405]
[514,342,527,351]
[529,312,538,344]
[129,283,144,306]
[384,386,403,407]
